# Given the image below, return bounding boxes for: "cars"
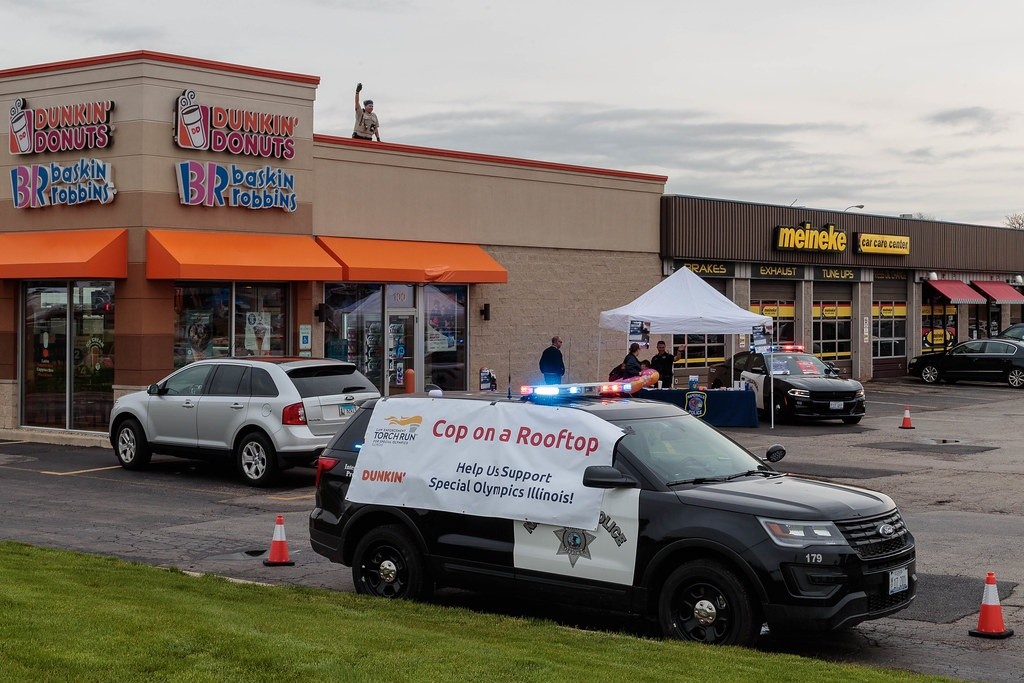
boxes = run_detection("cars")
[923,318,999,337]
[907,338,1024,389]
[708,345,866,425]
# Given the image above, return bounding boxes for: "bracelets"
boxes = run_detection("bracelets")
[678,350,682,352]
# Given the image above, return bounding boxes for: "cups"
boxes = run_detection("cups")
[657,381,662,389]
[740,381,745,390]
[734,381,739,390]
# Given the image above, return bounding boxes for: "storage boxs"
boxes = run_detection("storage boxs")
[50,317,77,337]
[327,337,348,362]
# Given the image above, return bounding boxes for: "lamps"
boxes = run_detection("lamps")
[920,271,938,281]
[1005,274,1023,284]
[39,322,49,358]
[480,303,490,321]
[842,205,864,213]
[823,222,835,231]
[313,303,328,323]
[800,220,812,229]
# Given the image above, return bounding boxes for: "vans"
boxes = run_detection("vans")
[994,323,1024,340]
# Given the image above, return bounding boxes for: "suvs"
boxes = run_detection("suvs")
[109,355,382,487]
[309,381,919,649]
[174,334,287,363]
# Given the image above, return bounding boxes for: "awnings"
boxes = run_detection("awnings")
[146,229,342,280]
[0,228,128,278]
[970,281,1024,304]
[315,236,508,282]
[925,280,986,304]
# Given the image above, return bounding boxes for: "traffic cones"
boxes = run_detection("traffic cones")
[898,406,915,429]
[968,572,1014,638]
[263,514,296,566]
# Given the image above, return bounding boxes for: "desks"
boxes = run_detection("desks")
[640,387,760,429]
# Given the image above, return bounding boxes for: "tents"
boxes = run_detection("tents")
[337,283,464,315]
[597,266,773,428]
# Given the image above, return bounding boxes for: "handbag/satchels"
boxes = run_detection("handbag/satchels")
[609,363,626,381]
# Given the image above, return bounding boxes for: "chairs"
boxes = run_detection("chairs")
[754,357,768,370]
[787,358,804,375]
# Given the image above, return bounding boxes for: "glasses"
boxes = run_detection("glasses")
[558,341,562,343]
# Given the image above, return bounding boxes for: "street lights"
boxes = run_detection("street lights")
[844,204,864,213]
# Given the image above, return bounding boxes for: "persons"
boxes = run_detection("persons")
[352,83,380,141]
[651,341,686,388]
[539,336,565,384]
[609,343,651,381]
